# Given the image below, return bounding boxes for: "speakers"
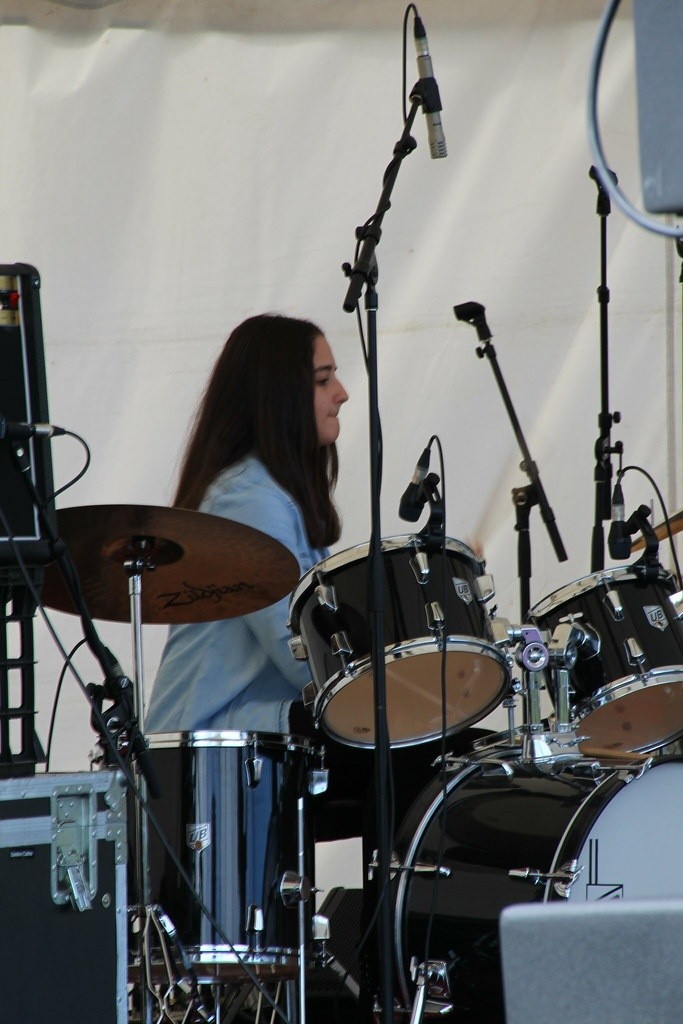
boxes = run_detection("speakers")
[499,900,683,1024]
[0,263,59,567]
[296,886,362,1024]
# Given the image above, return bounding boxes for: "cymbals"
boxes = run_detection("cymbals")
[36,505,299,623]
[631,511,682,552]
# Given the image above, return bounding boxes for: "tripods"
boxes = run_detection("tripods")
[122,560,201,1024]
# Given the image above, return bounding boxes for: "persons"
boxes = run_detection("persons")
[143,313,496,845]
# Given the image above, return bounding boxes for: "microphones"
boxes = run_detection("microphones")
[414,17,448,159]
[608,484,632,561]
[104,646,166,800]
[0,420,66,439]
[398,447,432,523]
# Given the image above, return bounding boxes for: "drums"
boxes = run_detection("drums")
[131,728,313,973]
[360,717,683,1023]
[290,533,512,749]
[525,563,682,752]
